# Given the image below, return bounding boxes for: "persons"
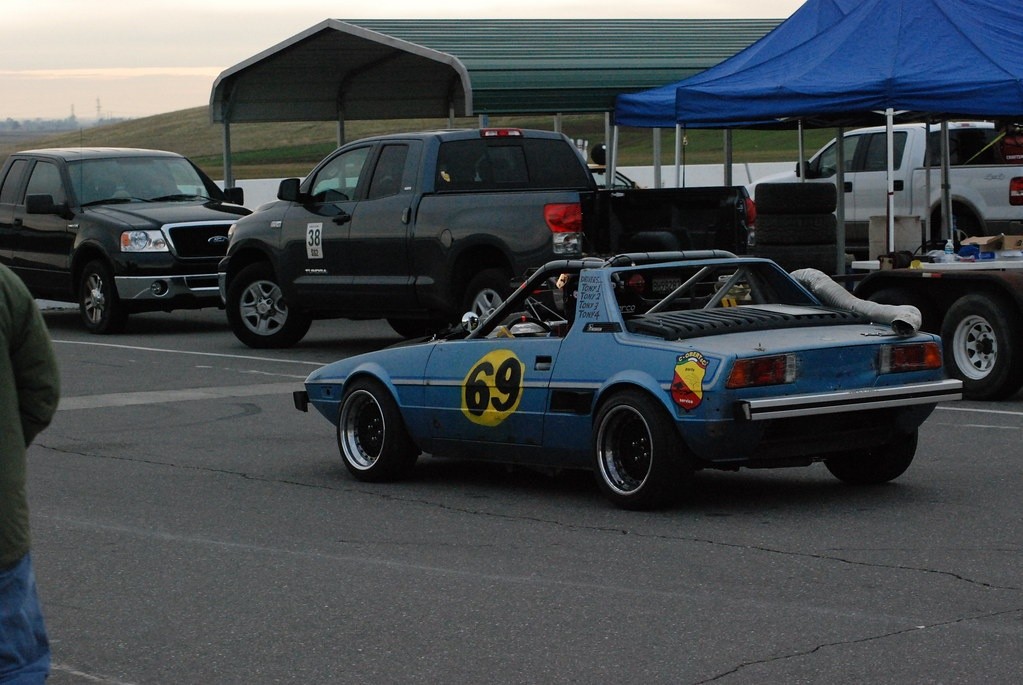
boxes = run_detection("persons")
[535,298,576,338]
[0,263,60,685]
[140,162,181,199]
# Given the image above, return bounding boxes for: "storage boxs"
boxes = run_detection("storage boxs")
[960,233,1023,251]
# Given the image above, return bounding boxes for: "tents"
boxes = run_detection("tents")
[612,1,1023,258]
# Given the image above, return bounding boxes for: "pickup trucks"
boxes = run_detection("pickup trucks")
[216,126,758,351]
[0,146,256,334]
[744,120,1023,238]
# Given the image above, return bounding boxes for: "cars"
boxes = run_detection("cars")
[291,249,967,516]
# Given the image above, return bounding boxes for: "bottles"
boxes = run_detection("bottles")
[945,239,953,263]
